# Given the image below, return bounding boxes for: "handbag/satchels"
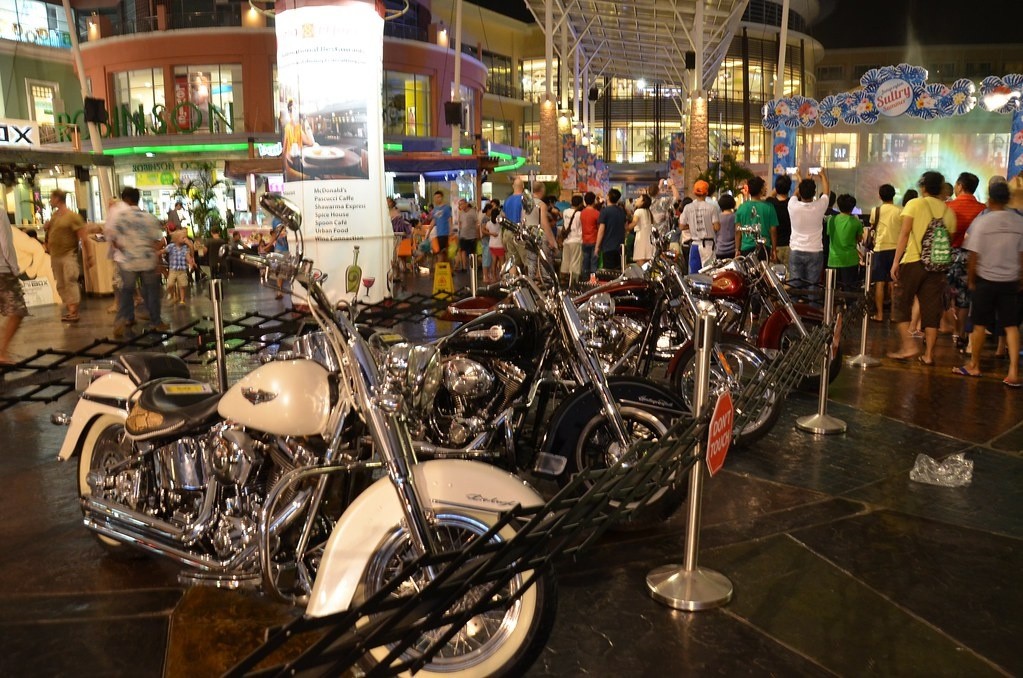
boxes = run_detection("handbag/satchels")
[560,225,572,241]
[867,206,880,250]
[447,240,458,261]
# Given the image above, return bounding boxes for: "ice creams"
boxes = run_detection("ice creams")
[183,237,192,246]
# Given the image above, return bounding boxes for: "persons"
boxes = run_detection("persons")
[273,213,291,299]
[104,185,197,336]
[0,182,28,364]
[192,225,269,277]
[43,190,95,322]
[387,167,1023,387]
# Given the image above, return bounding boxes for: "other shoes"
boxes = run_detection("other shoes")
[107,298,185,312]
[952,333,966,347]
[150,322,171,330]
[61,309,79,321]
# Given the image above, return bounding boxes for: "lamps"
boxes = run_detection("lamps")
[248,136,255,143]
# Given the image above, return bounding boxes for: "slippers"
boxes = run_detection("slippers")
[993,351,1006,358]
[1003,376,1021,386]
[918,355,934,365]
[960,349,972,357]
[952,366,983,377]
[870,315,883,322]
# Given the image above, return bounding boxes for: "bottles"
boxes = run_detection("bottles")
[187,224,193,238]
[303,110,366,139]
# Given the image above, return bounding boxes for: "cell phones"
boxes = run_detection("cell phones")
[786,167,798,174]
[808,167,821,175]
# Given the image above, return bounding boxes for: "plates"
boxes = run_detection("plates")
[303,147,345,160]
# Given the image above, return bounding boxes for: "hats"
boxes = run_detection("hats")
[694,180,709,196]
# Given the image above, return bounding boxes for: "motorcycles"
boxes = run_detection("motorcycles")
[350,217,848,540]
[50,192,556,678]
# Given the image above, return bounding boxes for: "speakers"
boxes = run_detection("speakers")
[444,102,462,125]
[74,165,90,182]
[686,51,695,69]
[83,97,106,123]
[588,88,598,100]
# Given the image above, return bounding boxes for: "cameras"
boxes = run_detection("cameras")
[663,180,668,185]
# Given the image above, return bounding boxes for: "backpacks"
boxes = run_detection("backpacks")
[919,218,954,273]
